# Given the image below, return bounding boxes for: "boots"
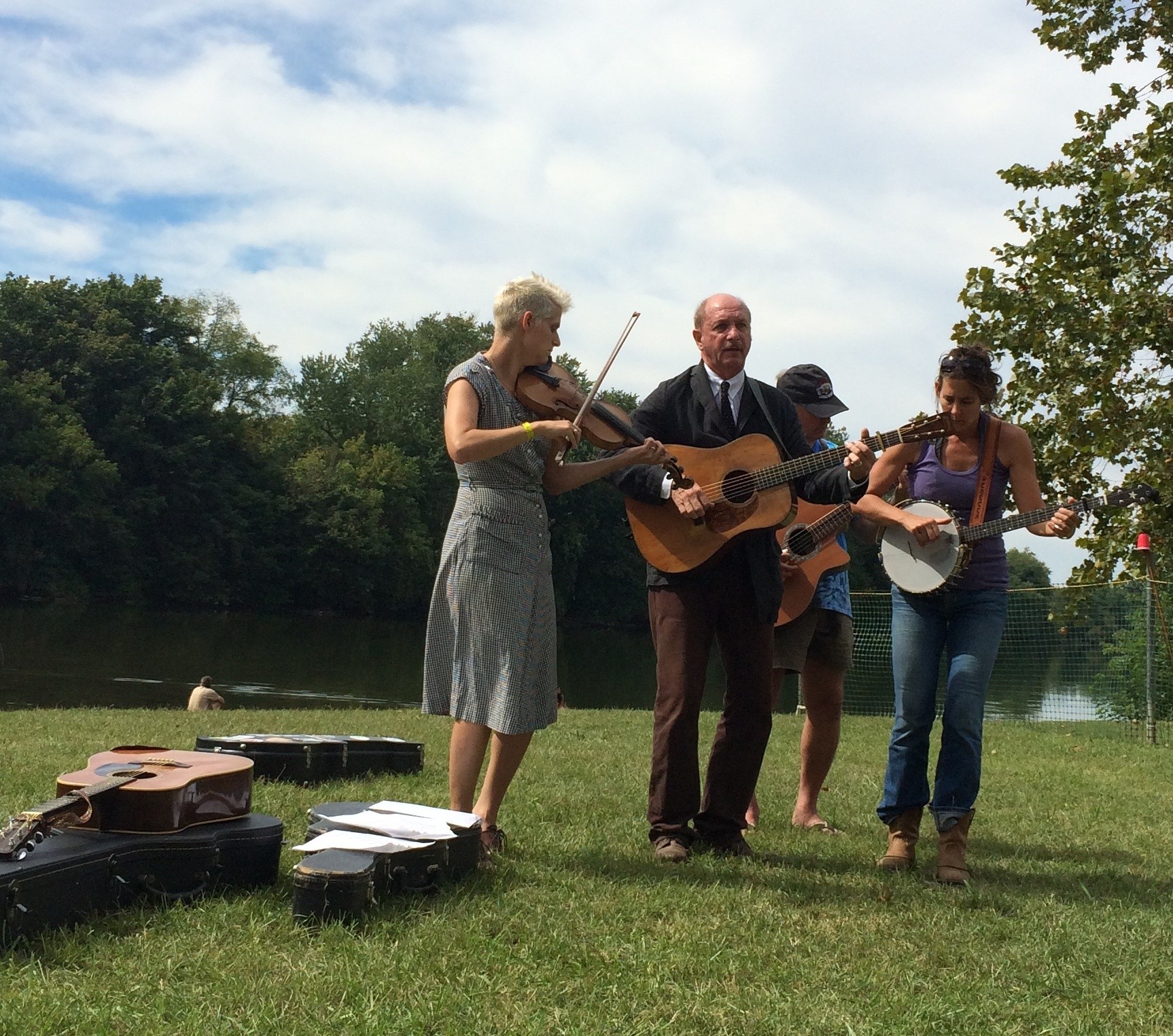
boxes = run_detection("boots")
[878,806,924,870]
[937,808,976,882]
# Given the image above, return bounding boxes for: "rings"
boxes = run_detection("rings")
[855,462,863,468]
[1053,524,1059,530]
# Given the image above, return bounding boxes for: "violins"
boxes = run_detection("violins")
[515,355,695,491]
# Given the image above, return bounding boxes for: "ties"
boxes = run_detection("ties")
[721,382,735,439]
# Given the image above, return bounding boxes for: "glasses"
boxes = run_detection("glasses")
[940,356,987,377]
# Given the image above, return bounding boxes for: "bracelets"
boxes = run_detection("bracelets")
[1056,527,1076,540]
[523,420,536,444]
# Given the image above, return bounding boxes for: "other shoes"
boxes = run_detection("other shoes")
[481,825,508,857]
[716,837,770,862]
[653,837,687,861]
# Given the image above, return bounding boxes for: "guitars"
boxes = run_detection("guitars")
[772,412,929,628]
[879,483,1154,594]
[0,745,256,861]
[622,409,957,573]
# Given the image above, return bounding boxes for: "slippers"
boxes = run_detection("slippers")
[806,820,838,837]
[742,823,757,837]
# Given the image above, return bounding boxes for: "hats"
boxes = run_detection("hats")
[777,364,849,418]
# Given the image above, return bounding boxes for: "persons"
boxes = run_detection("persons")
[598,295,876,863]
[744,365,910,838]
[419,276,669,863]
[187,676,225,712]
[850,347,1079,884]
